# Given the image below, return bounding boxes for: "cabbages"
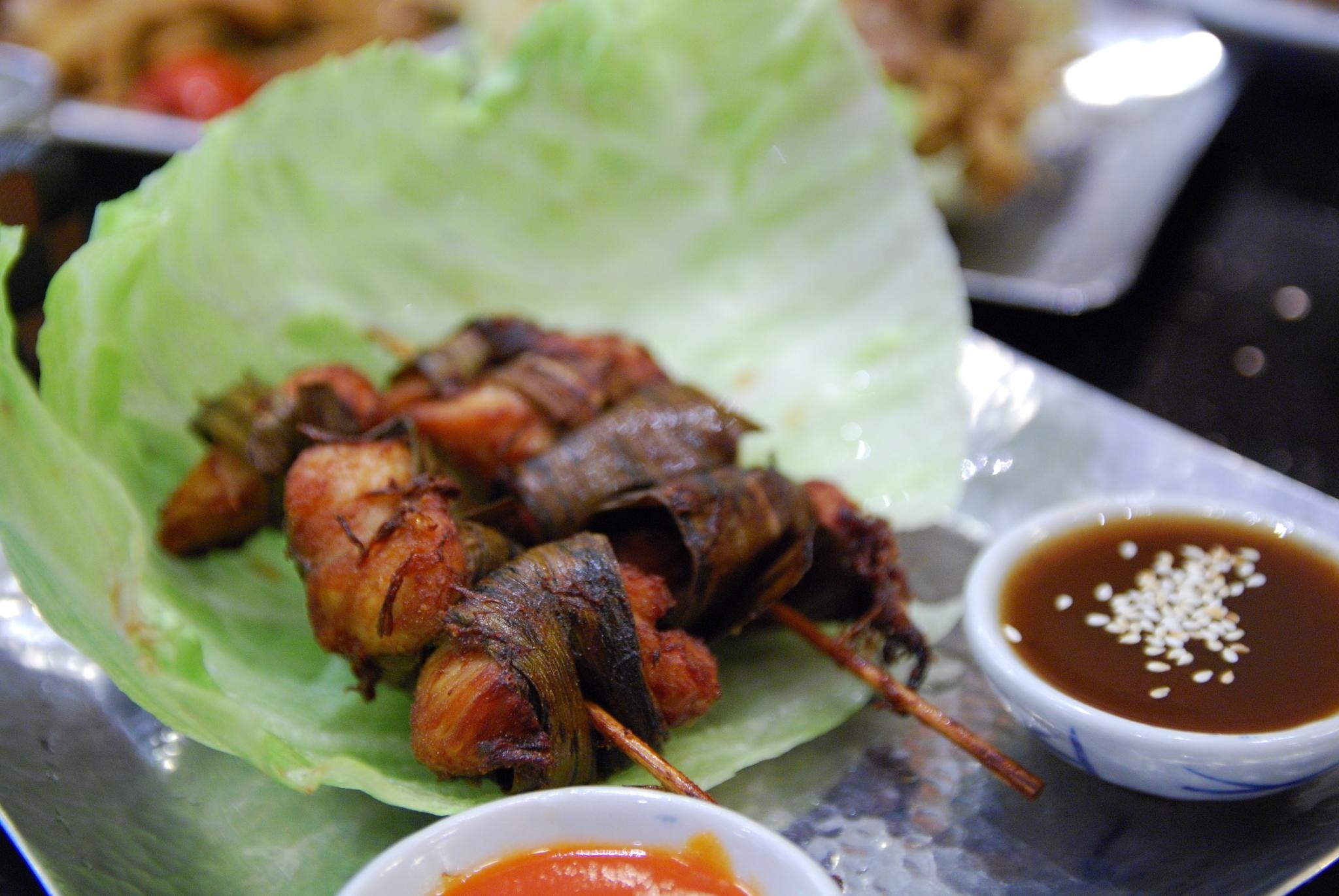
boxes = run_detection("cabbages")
[0,1,969,824]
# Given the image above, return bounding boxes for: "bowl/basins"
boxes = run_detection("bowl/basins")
[334,784,844,896]
[964,493,1338,802]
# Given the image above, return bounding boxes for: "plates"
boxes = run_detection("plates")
[1,311,1337,896]
[55,0,1247,321]
